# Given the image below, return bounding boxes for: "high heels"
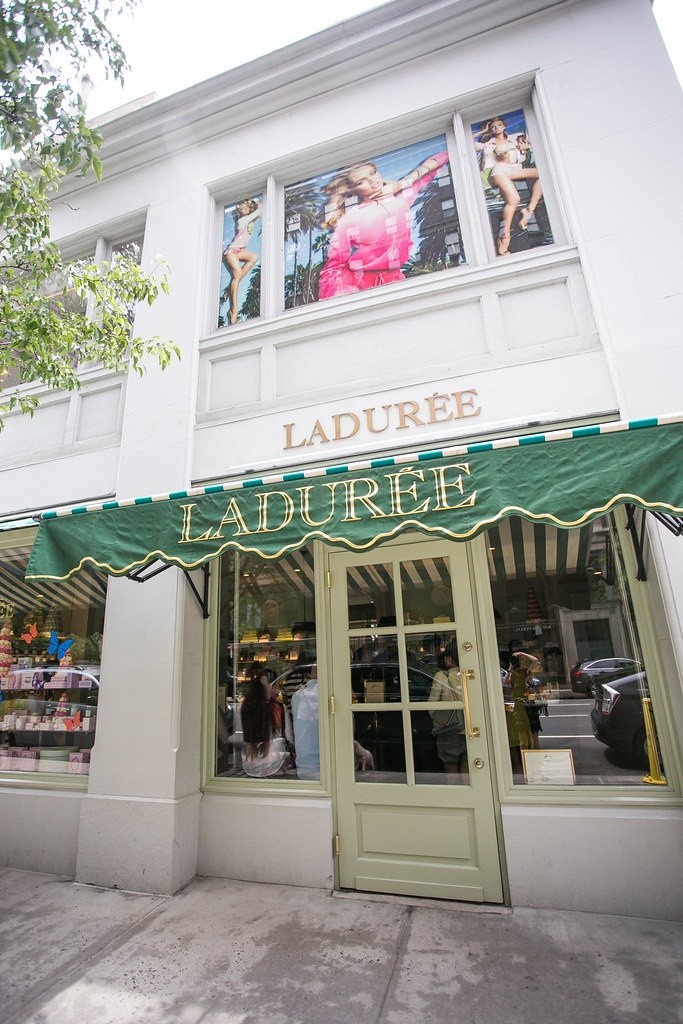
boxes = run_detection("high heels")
[516,208,535,231]
[220,286,230,302]
[496,236,511,256]
[226,309,236,325]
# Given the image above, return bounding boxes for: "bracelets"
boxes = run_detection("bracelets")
[507,670,511,672]
[416,170,419,179]
[420,165,430,172]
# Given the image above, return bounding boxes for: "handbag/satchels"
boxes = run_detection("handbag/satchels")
[431,670,466,737]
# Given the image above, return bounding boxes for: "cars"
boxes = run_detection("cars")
[570,658,643,698]
[0,664,100,749]
[591,662,663,772]
[225,652,540,783]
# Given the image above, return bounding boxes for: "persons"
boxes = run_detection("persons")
[473,116,542,255]
[215,651,373,780]
[318,151,448,300]
[223,200,262,324]
[428,641,469,785]
[504,652,537,769]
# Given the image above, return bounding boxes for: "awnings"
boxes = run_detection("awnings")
[0,544,108,613]
[271,513,593,599]
[24,413,683,619]
